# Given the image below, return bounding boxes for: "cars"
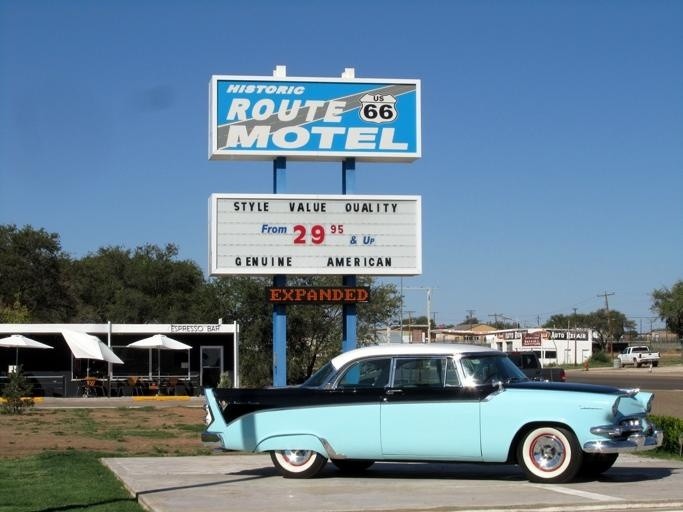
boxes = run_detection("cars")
[200,341,666,484]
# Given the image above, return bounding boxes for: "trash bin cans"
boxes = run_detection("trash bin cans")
[613,358,621,368]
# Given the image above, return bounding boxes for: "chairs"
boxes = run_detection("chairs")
[78,375,176,397]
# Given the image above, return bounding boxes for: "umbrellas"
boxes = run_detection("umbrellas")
[0,334,54,373]
[124,332,193,392]
[57,330,124,395]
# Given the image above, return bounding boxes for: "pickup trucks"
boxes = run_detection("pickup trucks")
[505,351,568,382]
[617,345,661,368]
